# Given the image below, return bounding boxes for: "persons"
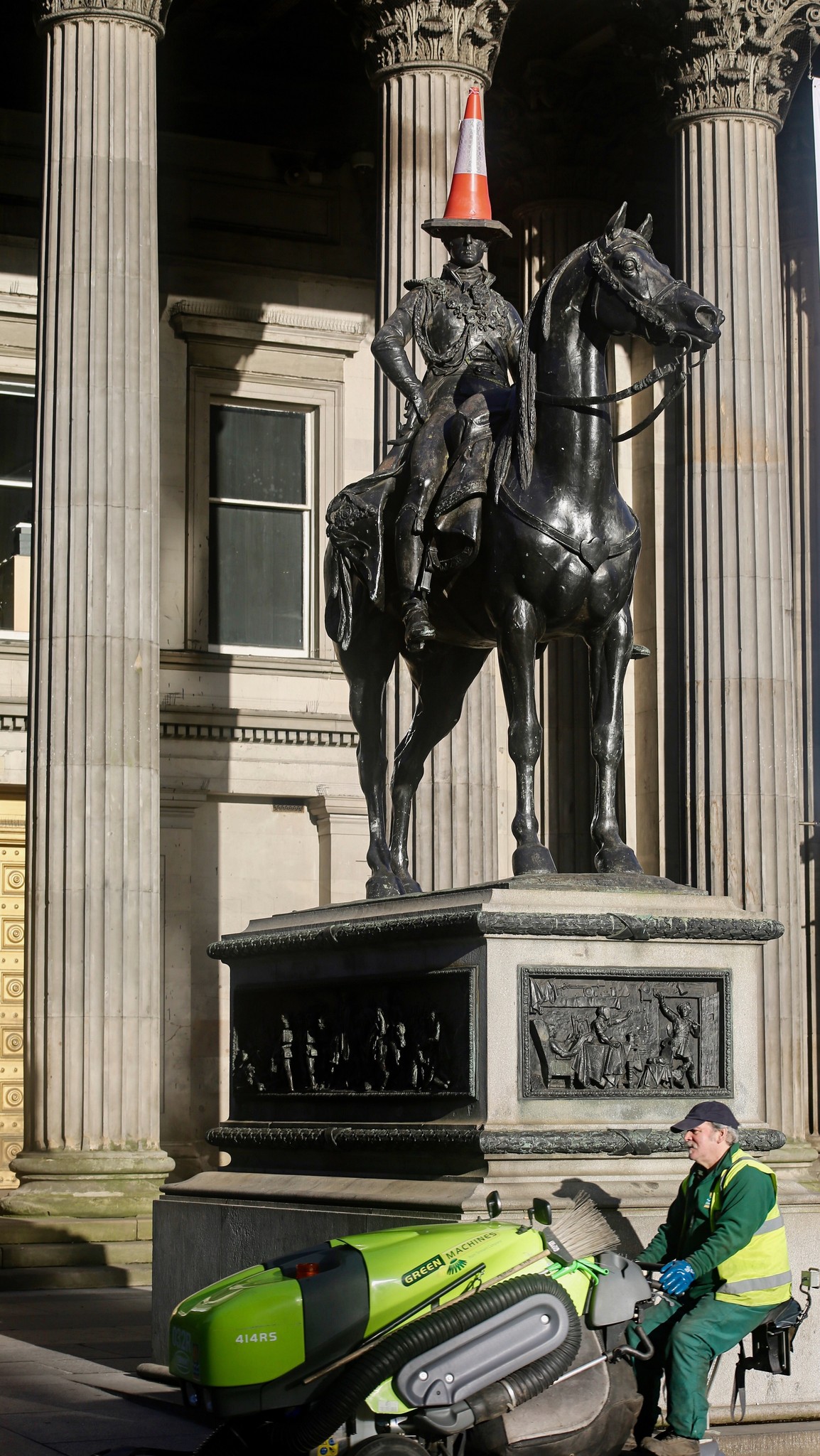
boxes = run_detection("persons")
[631,1101,791,1456]
[369,81,535,656]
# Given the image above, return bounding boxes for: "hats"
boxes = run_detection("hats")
[670,1101,738,1134]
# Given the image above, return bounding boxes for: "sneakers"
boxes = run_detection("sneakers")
[622,1428,651,1452]
[641,1431,701,1456]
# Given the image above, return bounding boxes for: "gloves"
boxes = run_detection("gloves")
[658,1260,695,1295]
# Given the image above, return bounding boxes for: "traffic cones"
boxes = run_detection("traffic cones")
[420,85,514,243]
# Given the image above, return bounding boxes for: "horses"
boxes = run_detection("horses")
[315,199,725,904]
